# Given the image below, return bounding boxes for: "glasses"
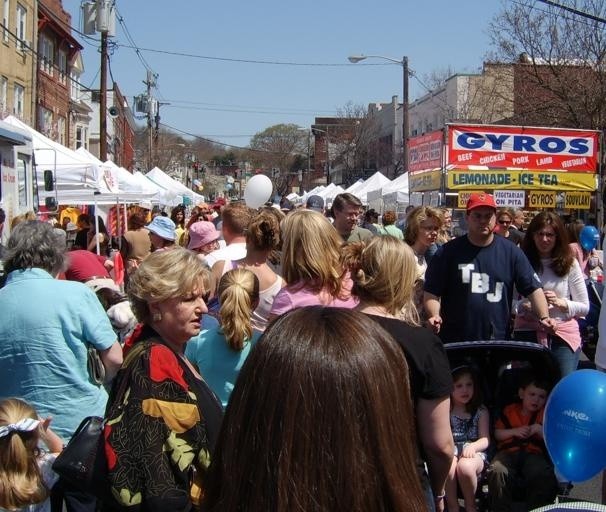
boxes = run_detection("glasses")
[538,232,554,236]
[499,220,511,224]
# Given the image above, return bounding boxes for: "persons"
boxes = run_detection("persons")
[268,209,361,327]
[103,245,223,512]
[422,192,558,342]
[199,307,428,512]
[444,364,493,512]
[0,220,123,452]
[486,365,549,512]
[184,269,264,411]
[0,192,604,349]
[0,397,67,512]
[512,212,590,378]
[345,235,453,512]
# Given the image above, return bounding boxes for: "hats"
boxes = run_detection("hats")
[466,191,497,211]
[188,221,220,250]
[306,195,324,208]
[144,216,178,241]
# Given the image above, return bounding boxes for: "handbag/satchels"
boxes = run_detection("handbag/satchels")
[52,344,159,499]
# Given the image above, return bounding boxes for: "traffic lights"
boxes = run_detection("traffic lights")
[194,161,199,179]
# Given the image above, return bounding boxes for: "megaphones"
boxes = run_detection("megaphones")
[108,106,120,118]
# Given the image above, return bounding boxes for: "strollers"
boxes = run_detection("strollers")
[442,340,557,505]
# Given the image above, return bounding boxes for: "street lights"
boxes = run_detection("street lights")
[346,55,410,174]
[296,125,331,184]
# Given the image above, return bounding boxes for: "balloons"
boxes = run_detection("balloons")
[580,226,600,252]
[244,174,273,210]
[194,179,203,191]
[543,369,606,483]
